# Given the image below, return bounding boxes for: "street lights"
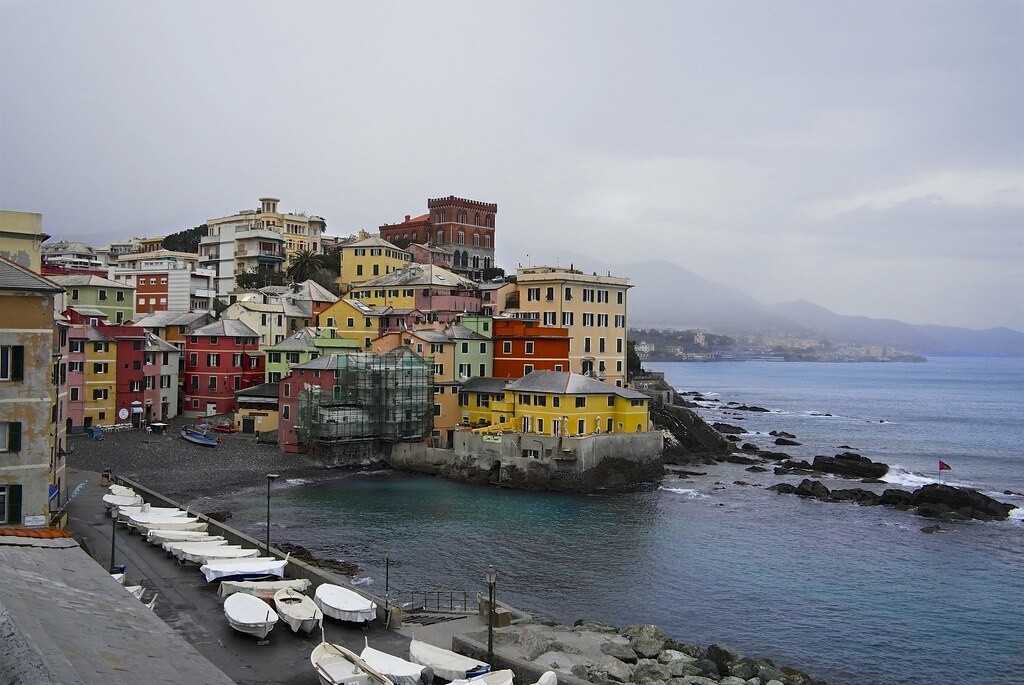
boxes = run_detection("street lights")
[267,474,280,556]
[484,565,497,670]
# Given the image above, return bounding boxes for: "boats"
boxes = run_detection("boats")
[197,418,213,424]
[181,425,218,448]
[221,579,309,605]
[124,585,146,601]
[316,583,377,625]
[410,635,491,679]
[209,420,237,433]
[103,483,275,565]
[360,636,434,685]
[111,572,125,585]
[200,552,290,584]
[224,592,278,638]
[274,588,323,632]
[447,669,513,685]
[310,627,394,685]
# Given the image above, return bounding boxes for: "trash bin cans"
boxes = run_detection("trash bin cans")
[87,426,102,439]
[494,607,511,628]
[100,472,109,486]
[103,467,112,481]
